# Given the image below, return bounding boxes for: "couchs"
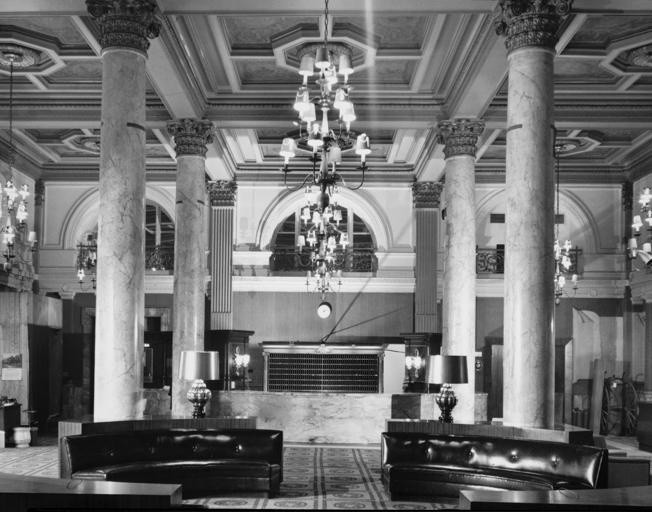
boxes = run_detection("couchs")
[378,430,610,503]
[56,424,284,500]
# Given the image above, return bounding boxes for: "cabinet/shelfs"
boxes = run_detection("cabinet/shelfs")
[1,404,24,449]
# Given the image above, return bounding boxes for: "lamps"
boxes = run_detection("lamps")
[225,351,251,384]
[406,348,425,382]
[621,183,652,269]
[550,143,584,305]
[0,51,43,272]
[177,349,221,418]
[75,241,98,284]
[422,353,469,423]
[278,1,375,298]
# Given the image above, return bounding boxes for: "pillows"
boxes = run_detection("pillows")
[159,431,241,460]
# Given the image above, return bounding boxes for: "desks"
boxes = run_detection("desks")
[383,417,595,446]
[55,416,265,478]
